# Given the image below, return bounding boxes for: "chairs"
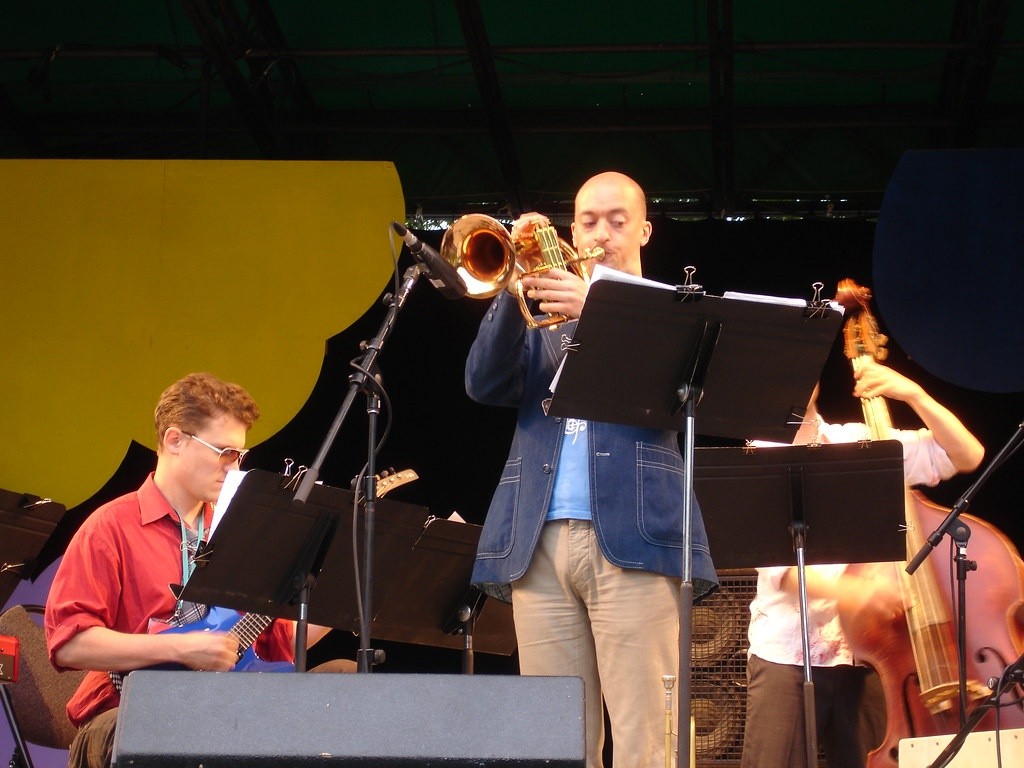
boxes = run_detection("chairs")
[0,604,91,768]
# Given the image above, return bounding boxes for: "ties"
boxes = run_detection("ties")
[176,529,209,626]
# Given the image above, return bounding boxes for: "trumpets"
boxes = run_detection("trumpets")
[439,211,607,331]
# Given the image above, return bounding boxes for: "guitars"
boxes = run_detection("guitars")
[145,464,421,673]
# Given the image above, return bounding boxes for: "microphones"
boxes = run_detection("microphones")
[392,221,468,300]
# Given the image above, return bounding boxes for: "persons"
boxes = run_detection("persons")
[740,362,985,768]
[464,171,720,768]
[44,373,357,768]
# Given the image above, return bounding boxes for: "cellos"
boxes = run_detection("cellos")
[824,276,1024,768]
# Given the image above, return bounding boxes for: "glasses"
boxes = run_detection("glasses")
[181,430,249,467]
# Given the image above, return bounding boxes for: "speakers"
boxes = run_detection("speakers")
[111,669,588,768]
